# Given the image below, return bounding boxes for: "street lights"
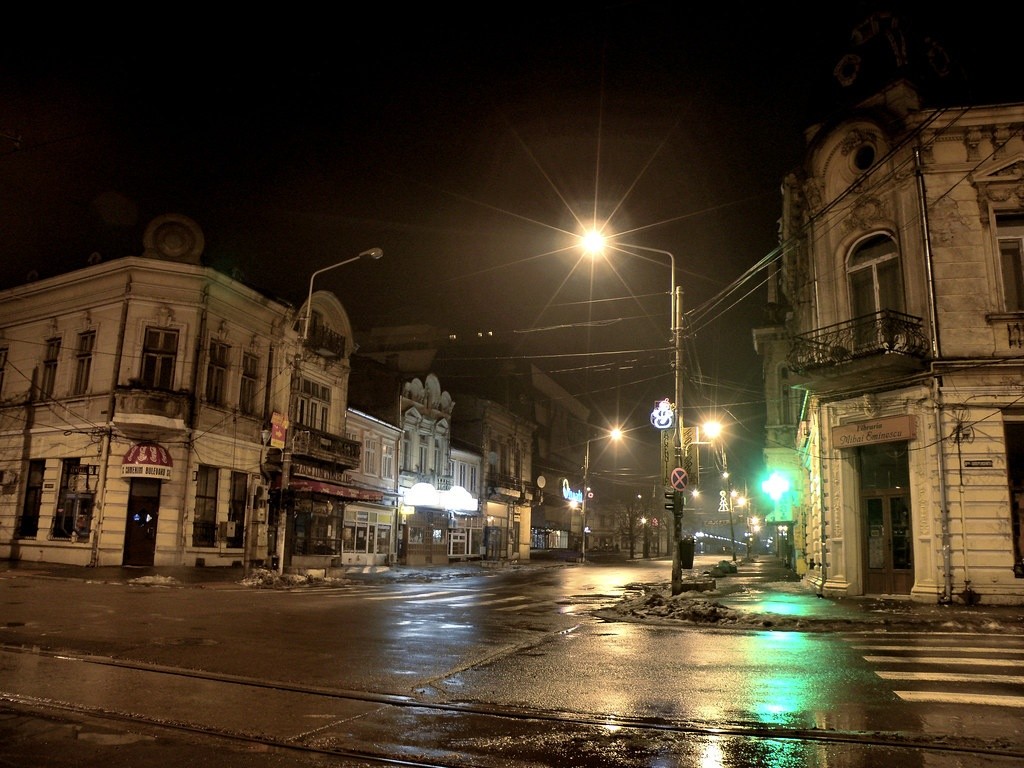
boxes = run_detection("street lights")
[586,233,684,591]
[275,247,384,576]
[581,431,621,563]
[707,420,738,563]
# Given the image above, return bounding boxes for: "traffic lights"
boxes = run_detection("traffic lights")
[282,488,293,511]
[664,489,685,518]
[268,488,277,506]
